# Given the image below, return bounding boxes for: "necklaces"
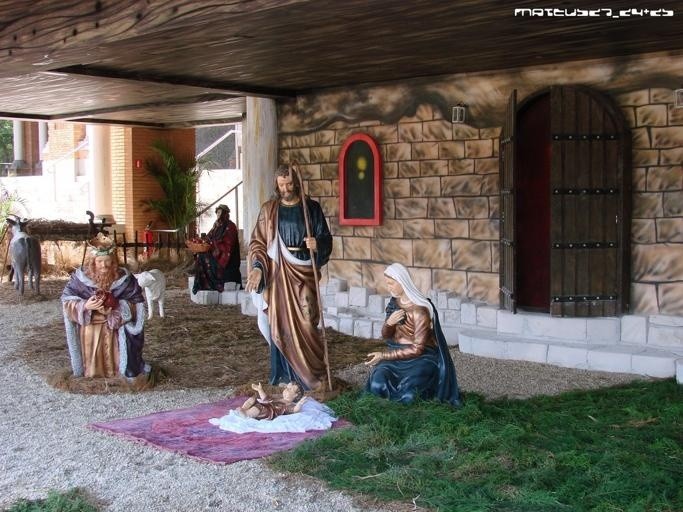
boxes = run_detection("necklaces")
[399,296,412,306]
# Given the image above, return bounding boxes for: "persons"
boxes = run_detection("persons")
[363,263,456,406]
[189,204,243,294]
[59,232,152,385]
[233,379,307,422]
[242,162,333,394]
